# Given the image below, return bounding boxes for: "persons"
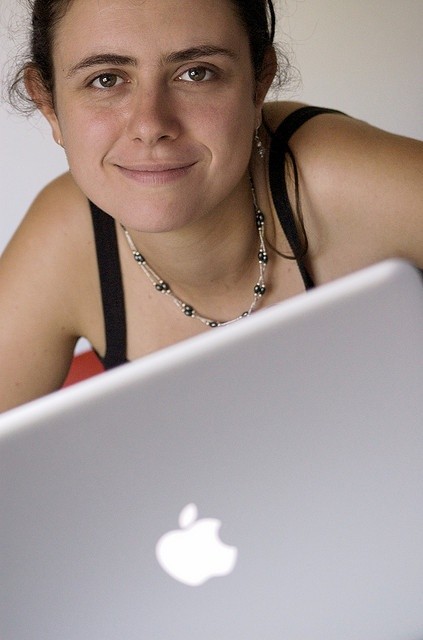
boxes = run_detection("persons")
[0,2,422,416]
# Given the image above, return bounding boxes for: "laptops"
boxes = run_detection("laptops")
[1,256,423,640]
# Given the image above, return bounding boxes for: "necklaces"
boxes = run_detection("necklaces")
[120,165,268,329]
